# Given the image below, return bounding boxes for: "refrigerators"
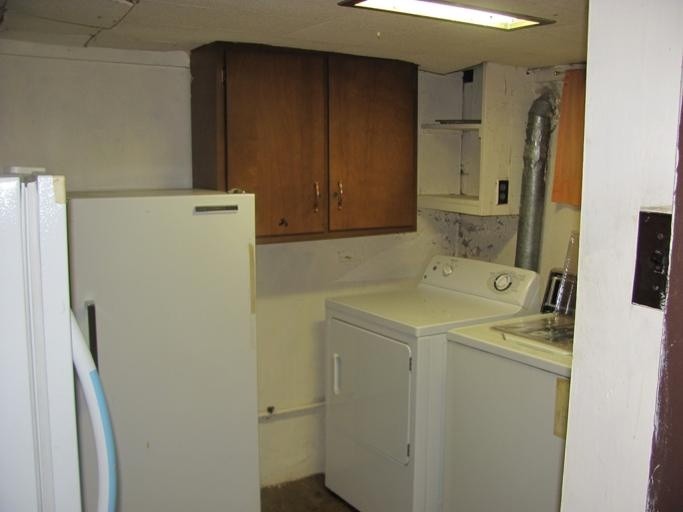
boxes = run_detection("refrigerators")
[66,188,262,509]
[0,170,118,509]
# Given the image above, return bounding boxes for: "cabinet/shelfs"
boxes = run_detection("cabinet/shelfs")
[417,62,532,216]
[189,41,419,245]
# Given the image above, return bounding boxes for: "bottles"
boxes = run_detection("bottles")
[548,230,578,326]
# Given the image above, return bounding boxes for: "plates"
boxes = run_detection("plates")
[432,119,481,125]
